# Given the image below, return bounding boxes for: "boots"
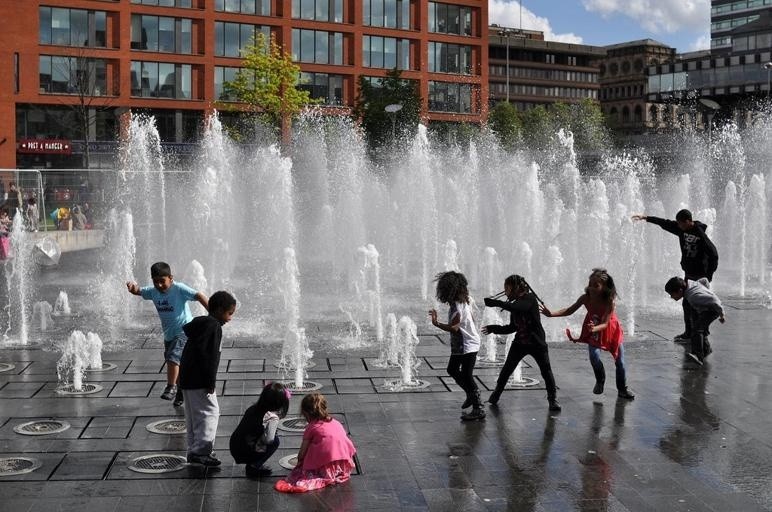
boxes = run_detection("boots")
[460,389,486,420]
[456,377,472,410]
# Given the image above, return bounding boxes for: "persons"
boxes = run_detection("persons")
[272,393,356,492]
[664,276,726,366]
[229,382,290,475]
[482,274,561,411]
[428,271,487,422]
[0,175,98,260]
[176,290,236,466]
[631,208,718,340]
[126,262,209,406]
[539,269,635,400]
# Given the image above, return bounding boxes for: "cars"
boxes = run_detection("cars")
[18,184,41,200]
[48,185,76,203]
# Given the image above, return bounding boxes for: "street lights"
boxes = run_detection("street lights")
[674,104,698,138]
[698,98,719,163]
[384,103,403,152]
[760,61,772,107]
[495,25,524,104]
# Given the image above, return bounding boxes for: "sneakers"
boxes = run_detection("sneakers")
[173,389,183,406]
[190,454,221,467]
[187,450,216,465]
[549,400,561,410]
[246,467,271,476]
[673,332,689,342]
[593,378,605,394]
[689,351,704,366]
[488,392,500,405]
[160,384,177,400]
[618,386,635,398]
